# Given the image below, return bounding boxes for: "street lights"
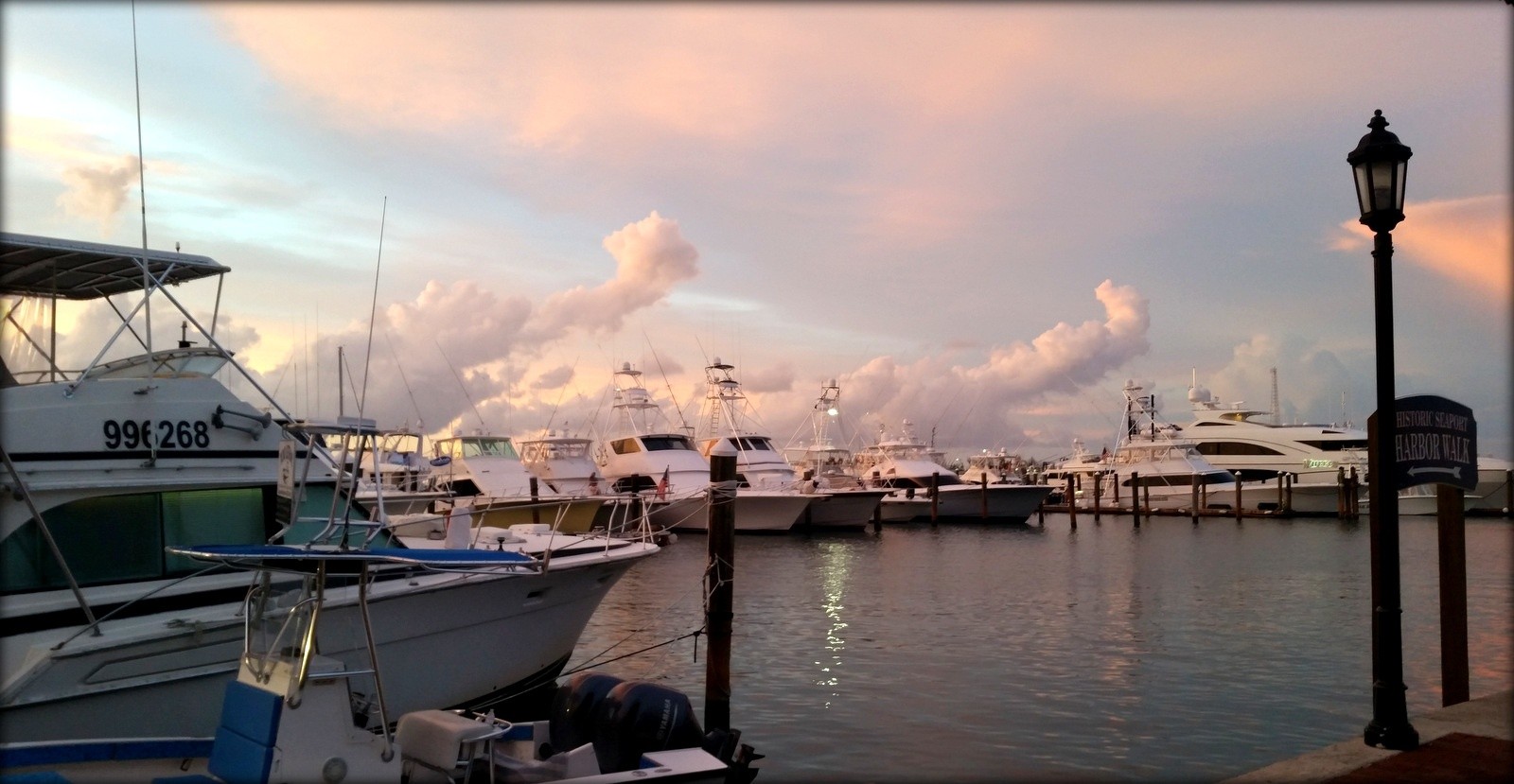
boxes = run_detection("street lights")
[1347,107,1421,746]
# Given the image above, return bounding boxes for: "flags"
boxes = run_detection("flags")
[657,468,668,501]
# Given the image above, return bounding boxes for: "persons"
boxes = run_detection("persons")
[588,472,598,495]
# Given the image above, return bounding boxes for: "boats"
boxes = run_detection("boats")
[288,350,1512,526]
[2,230,661,739]
[1,568,769,782]
